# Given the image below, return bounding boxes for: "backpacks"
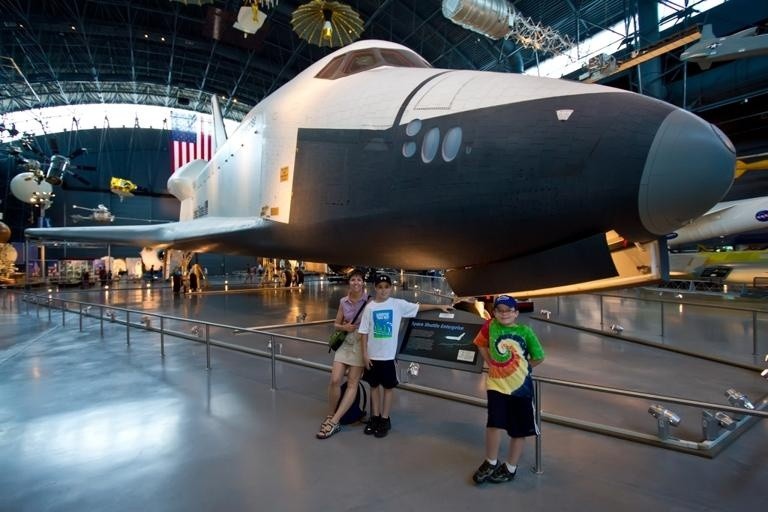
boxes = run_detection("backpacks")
[335,380,366,424]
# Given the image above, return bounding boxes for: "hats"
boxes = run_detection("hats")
[494,295,516,308]
[375,275,391,286]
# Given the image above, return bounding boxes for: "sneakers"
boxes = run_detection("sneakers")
[473,459,517,483]
[364,414,391,437]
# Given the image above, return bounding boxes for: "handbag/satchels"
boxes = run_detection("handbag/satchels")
[328,330,347,353]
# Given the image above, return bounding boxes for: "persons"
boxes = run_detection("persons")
[142,264,162,282]
[173,269,197,296]
[358,275,456,437]
[316,270,375,439]
[244,264,259,284]
[472,295,544,484]
[81,266,112,289]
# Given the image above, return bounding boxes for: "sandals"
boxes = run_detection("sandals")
[316,415,340,438]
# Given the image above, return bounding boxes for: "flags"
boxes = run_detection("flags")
[170,110,213,174]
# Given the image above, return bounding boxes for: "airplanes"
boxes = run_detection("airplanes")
[23,39,768,301]
